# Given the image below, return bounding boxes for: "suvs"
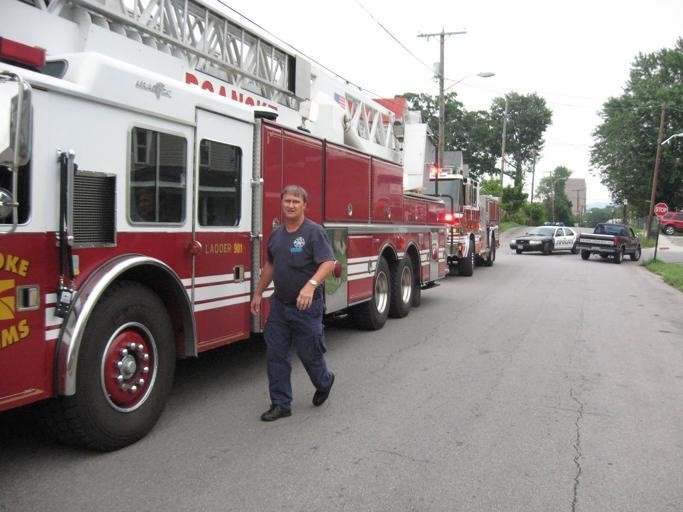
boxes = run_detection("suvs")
[657,211,683,235]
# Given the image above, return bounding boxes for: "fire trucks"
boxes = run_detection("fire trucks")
[1,0,452,453]
[428,148,501,275]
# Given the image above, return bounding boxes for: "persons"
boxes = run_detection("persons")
[248,184,338,421]
[132,187,172,222]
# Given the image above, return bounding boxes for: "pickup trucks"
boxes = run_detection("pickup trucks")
[580,223,641,262]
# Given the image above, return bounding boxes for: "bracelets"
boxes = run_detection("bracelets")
[308,279,319,287]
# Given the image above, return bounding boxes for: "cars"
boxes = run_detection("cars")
[510,225,579,255]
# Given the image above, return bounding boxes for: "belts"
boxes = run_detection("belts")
[274,292,319,306]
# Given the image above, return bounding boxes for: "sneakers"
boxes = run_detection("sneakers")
[261,403,291,421]
[313,371,335,406]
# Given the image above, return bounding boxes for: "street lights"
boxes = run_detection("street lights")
[645,132,682,239]
[437,72,495,168]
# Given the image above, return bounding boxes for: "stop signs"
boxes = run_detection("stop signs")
[654,204,669,217]
